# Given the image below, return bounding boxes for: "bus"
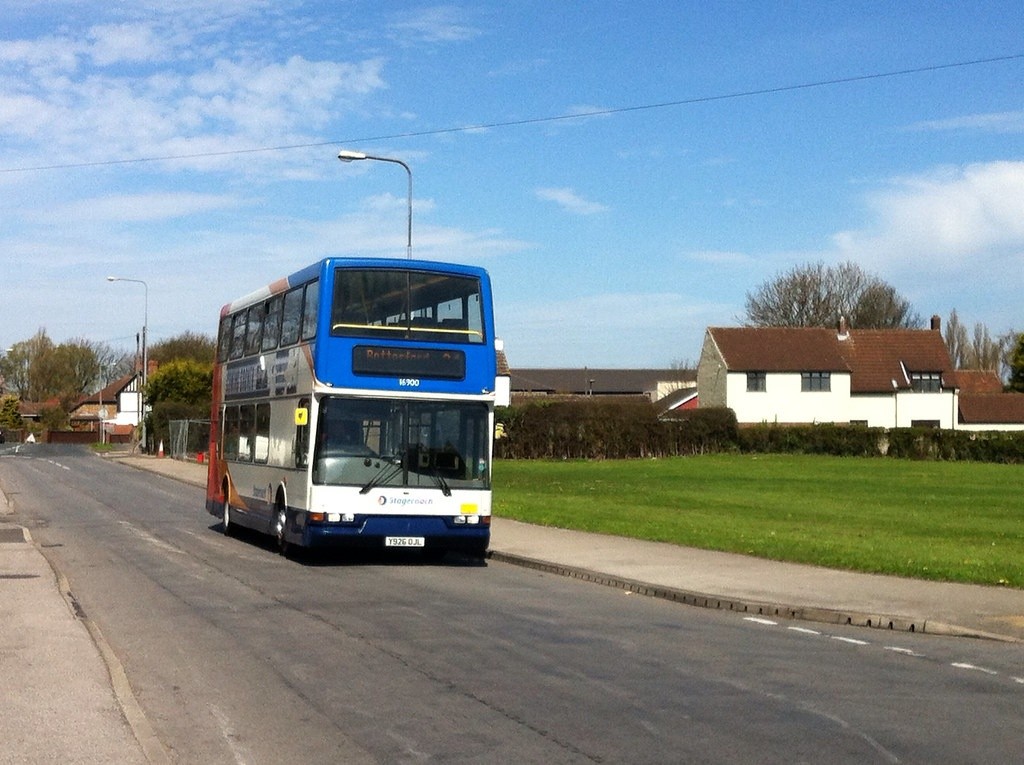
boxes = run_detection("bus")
[204,257,510,563]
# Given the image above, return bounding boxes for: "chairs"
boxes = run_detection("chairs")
[390,318,470,343]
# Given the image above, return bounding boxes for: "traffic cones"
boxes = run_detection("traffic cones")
[157,440,165,458]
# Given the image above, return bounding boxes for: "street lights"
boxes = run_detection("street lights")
[5,349,27,399]
[98,363,117,443]
[337,151,413,257]
[107,275,147,452]
